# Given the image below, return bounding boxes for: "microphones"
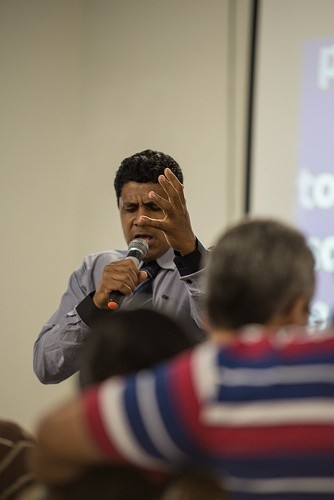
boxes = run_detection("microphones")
[105,238,149,311]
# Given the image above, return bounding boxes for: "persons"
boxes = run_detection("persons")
[31,219,334,500]
[33,148,214,384]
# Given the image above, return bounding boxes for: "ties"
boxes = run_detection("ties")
[132,261,160,310]
